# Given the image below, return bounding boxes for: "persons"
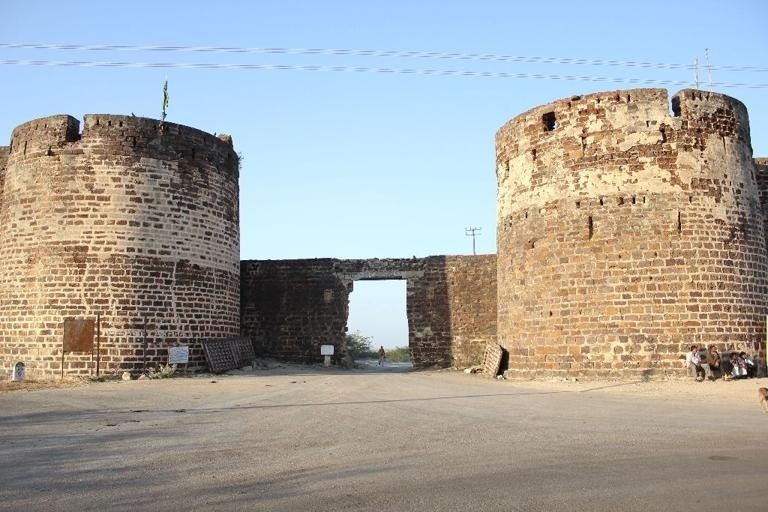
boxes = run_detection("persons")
[377,346,385,365]
[686,344,755,382]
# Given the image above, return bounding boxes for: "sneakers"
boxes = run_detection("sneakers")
[695,374,736,383]
